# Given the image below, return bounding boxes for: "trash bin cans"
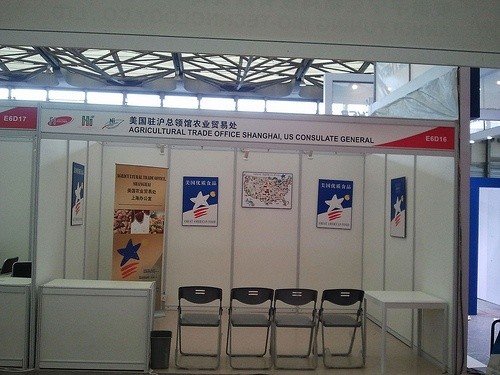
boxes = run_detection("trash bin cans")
[150,330,172,368]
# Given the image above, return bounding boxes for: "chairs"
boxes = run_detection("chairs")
[1,256,19,275]
[174,285,224,370]
[270,288,319,372]
[226,286,274,371]
[12,261,32,279]
[311,288,368,370]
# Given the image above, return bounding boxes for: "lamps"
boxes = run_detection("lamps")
[157,142,166,155]
[303,149,314,160]
[240,146,249,161]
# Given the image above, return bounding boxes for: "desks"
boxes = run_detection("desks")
[363,290,449,373]
[0,271,32,369]
[34,277,156,373]
